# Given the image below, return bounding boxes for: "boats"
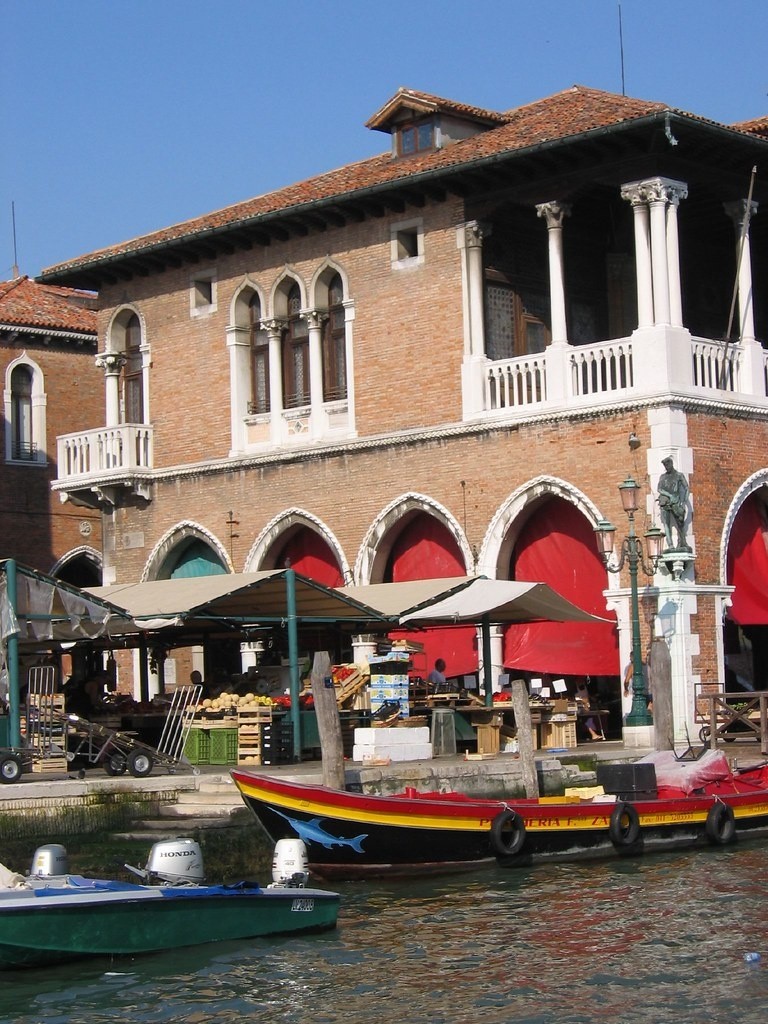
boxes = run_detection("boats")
[0,840,340,966]
[227,764,767,882]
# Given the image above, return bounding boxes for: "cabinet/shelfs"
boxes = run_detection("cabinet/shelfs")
[407,651,429,697]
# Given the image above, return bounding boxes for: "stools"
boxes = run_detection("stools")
[667,720,708,761]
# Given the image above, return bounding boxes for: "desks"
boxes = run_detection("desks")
[578,710,611,741]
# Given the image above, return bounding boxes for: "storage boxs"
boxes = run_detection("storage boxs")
[539,785,617,804]
[331,665,363,701]
[89,715,122,729]
[367,652,410,718]
[29,693,67,774]
[182,705,294,766]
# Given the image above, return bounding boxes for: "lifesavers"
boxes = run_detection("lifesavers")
[488,811,527,856]
[607,802,641,848]
[703,802,735,846]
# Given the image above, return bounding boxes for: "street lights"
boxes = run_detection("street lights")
[592,476,667,747]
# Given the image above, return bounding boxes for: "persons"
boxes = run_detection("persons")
[624,651,654,715]
[57,671,98,719]
[581,696,602,740]
[657,457,691,549]
[427,658,446,683]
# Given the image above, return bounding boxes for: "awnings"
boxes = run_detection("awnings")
[0,558,618,763]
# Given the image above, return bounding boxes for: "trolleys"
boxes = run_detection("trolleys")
[1,665,70,785]
[57,684,203,779]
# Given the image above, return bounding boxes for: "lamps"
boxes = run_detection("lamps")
[628,432,641,448]
